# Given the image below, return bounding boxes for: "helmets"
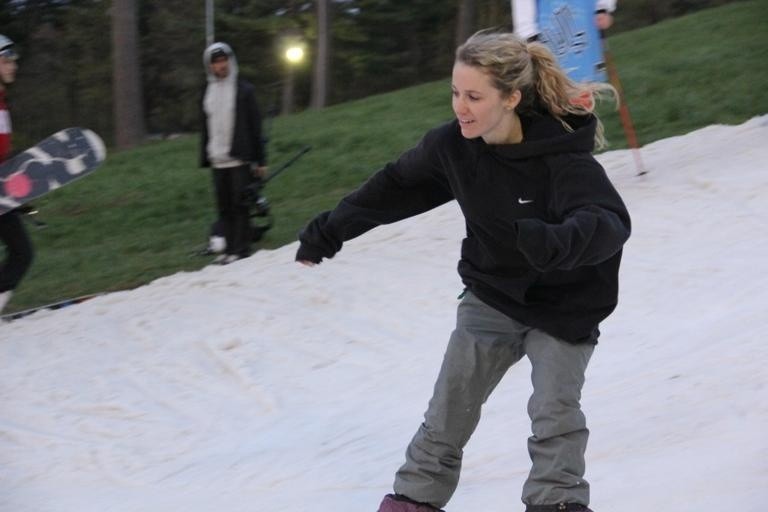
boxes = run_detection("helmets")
[0,33,18,62]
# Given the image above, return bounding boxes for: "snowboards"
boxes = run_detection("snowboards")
[0,126,108,215]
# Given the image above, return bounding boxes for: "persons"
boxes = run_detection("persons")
[195,38,272,268]
[0,33,38,316]
[507,1,622,112]
[291,26,633,511]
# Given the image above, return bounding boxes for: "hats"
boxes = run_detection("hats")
[210,49,234,61]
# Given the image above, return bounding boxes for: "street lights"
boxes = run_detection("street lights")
[280,36,305,113]
